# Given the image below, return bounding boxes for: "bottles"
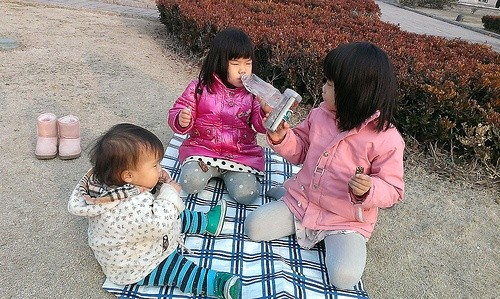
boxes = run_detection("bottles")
[241,73,281,108]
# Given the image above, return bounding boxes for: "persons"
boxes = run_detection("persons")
[244,42,406,290]
[67,123,242,299]
[168,29,271,204]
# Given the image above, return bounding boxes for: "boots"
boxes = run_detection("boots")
[58,115,82,159]
[35,113,57,159]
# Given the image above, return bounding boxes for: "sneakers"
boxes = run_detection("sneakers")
[205,199,227,236]
[213,271,241,299]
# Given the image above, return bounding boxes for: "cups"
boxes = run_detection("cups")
[264,88,303,134]
